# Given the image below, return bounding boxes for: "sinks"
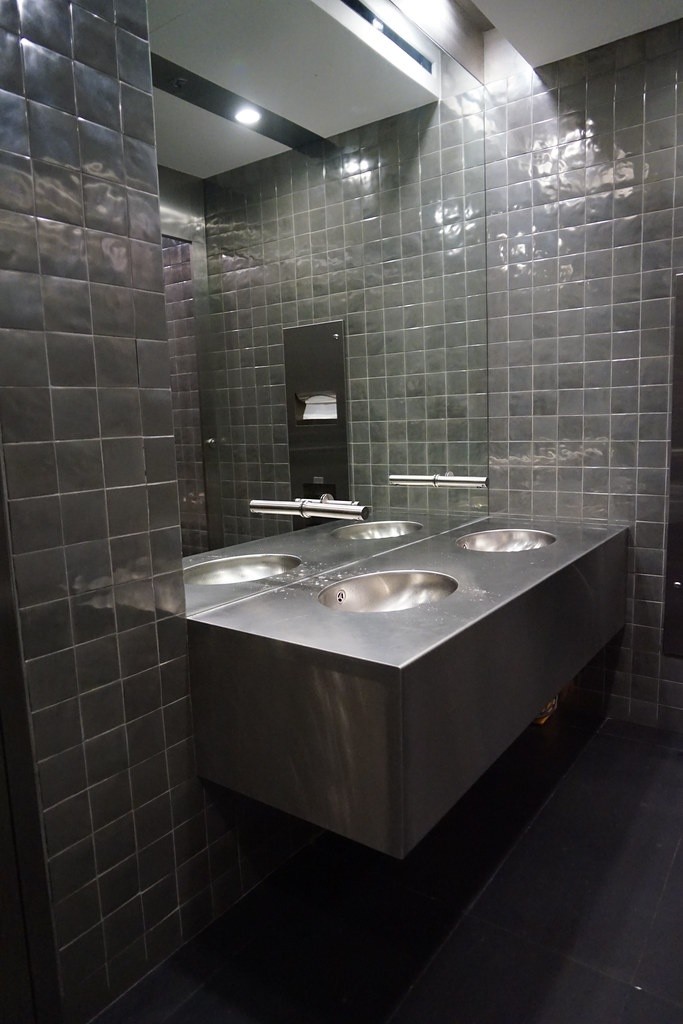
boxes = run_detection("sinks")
[331,520,425,539]
[184,554,302,585]
[456,527,558,553]
[317,569,459,612]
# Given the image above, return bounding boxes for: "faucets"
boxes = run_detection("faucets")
[249,499,303,518]
[435,473,489,489]
[388,475,435,486]
[303,502,369,521]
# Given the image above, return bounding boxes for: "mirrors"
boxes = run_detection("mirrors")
[145,0,490,619]
